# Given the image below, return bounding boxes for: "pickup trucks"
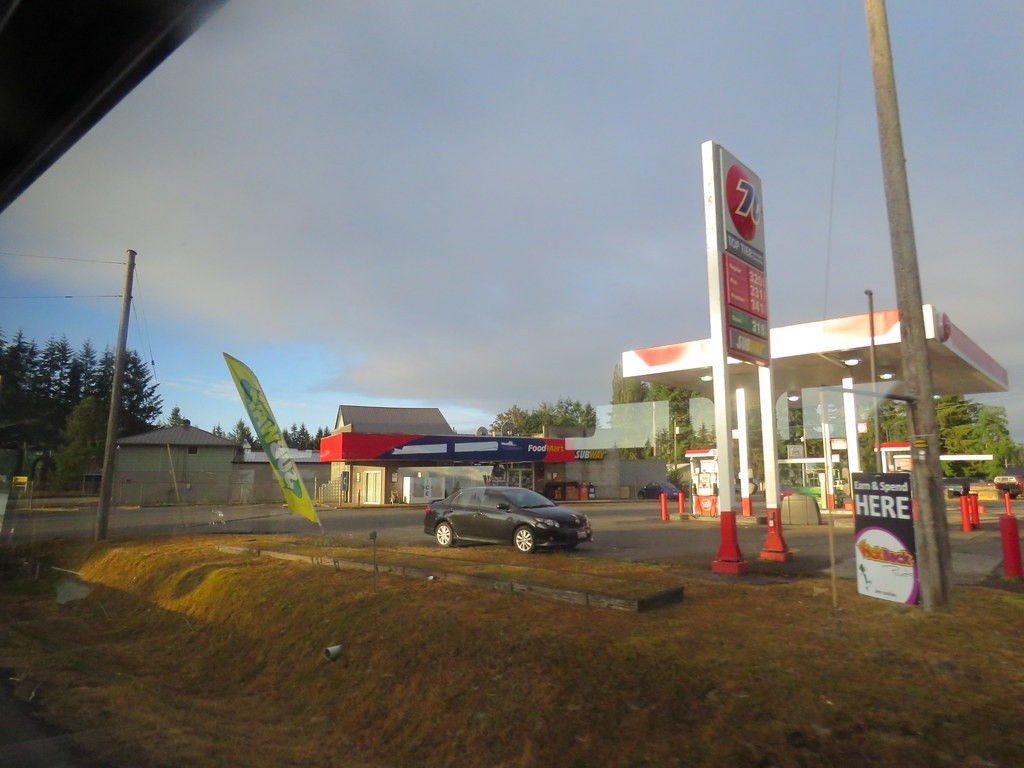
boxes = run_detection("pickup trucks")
[943,484,969,496]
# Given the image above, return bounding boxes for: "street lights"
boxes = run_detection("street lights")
[865,290,883,475]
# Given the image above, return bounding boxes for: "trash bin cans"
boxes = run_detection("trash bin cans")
[545,480,596,501]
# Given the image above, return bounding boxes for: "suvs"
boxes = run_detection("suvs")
[995,476,1024,499]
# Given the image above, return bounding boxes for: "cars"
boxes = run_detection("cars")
[637,484,683,500]
[421,486,593,554]
